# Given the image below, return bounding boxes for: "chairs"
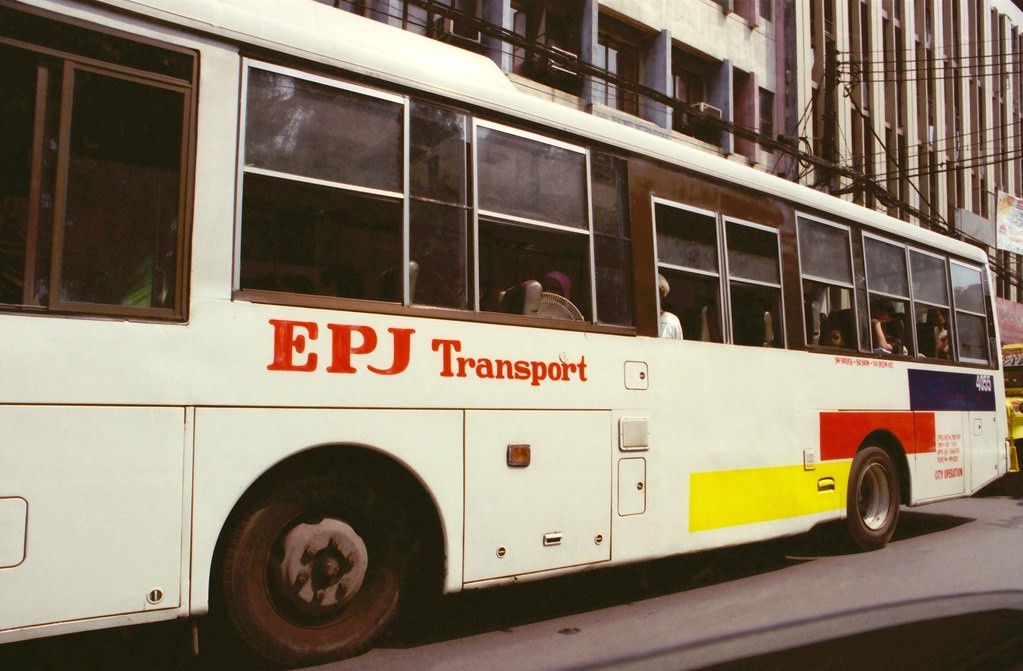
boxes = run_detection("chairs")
[366,261,542,318]
[674,299,940,358]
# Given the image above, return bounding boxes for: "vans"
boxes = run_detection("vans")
[1001,343,1023,483]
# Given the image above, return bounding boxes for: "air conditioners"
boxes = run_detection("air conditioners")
[436,17,481,49]
[686,101,723,134]
[538,45,577,82]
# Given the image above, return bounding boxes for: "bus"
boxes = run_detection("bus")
[0,0,1011,667]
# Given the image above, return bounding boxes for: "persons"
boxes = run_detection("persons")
[68,207,170,308]
[824,325,850,346]
[928,309,948,359]
[873,300,906,354]
[542,270,571,301]
[658,273,683,339]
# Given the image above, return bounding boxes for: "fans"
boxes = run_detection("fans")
[538,293,585,321]
